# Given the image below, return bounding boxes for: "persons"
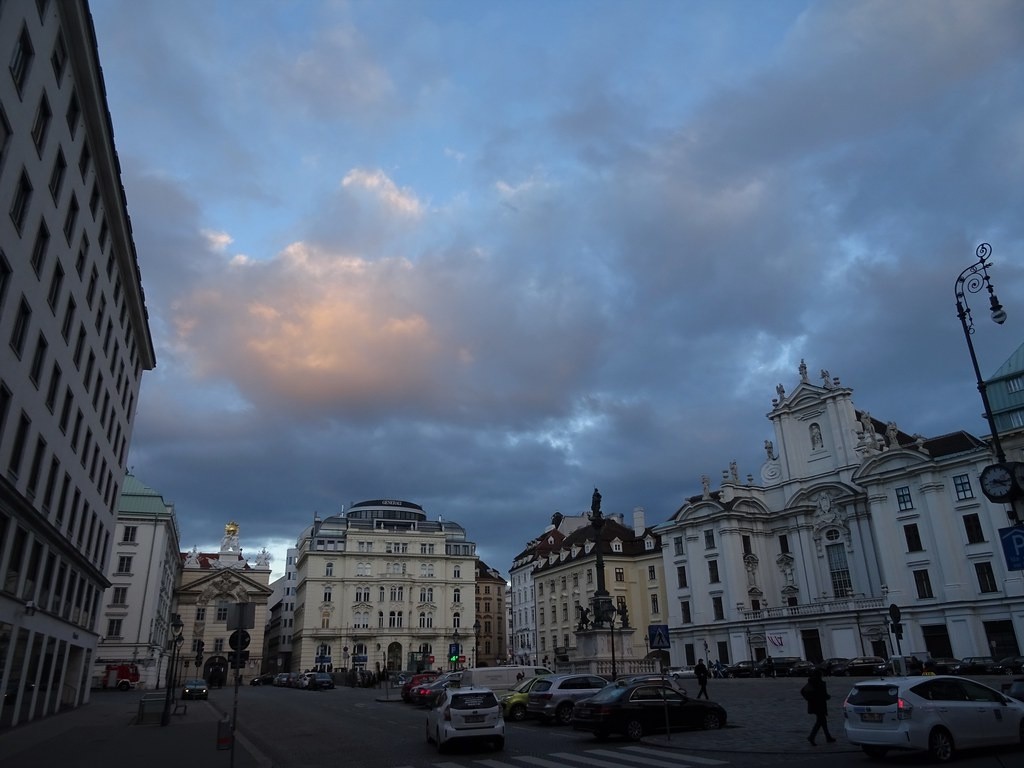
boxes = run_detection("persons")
[305,666,323,673]
[221,532,240,550]
[730,462,738,480]
[591,487,602,516]
[908,656,937,671]
[767,655,772,674]
[776,384,785,400]
[886,421,897,444]
[807,667,836,745]
[517,672,524,682]
[861,410,877,442]
[799,360,808,379]
[695,659,709,700]
[821,369,833,387]
[709,660,724,678]
[764,440,774,459]
[437,667,443,676]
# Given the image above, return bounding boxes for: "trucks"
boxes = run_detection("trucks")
[92,664,140,690]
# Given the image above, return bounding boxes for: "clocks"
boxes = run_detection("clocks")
[979,462,1016,504]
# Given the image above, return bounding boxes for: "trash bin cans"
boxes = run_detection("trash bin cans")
[891,655,906,676]
[216,719,232,750]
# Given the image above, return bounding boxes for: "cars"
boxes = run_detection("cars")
[401,669,465,706]
[843,676,1024,765]
[272,670,335,691]
[183,678,209,700]
[620,676,689,697]
[249,673,275,686]
[527,673,613,727]
[924,655,1000,676]
[887,654,922,676]
[722,660,760,678]
[572,686,729,744]
[499,675,556,722]
[669,666,698,679]
[425,686,506,754]
[992,655,1023,675]
[819,657,852,675]
[836,655,889,677]
[786,660,816,677]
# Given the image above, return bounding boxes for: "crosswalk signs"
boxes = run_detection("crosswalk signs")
[647,624,671,650]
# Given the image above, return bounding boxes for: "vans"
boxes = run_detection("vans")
[462,664,556,698]
[753,656,802,678]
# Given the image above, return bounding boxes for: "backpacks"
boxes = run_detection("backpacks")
[801,683,813,701]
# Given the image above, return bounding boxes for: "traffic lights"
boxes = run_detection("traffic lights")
[450,656,458,662]
[896,623,907,641]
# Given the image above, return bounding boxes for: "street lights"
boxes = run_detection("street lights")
[161,614,183,727]
[472,618,483,669]
[172,631,185,704]
[745,626,754,661]
[954,243,1024,536]
[452,628,460,672]
[587,486,626,629]
[607,603,618,679]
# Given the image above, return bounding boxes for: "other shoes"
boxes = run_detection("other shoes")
[807,736,817,746]
[827,737,836,742]
